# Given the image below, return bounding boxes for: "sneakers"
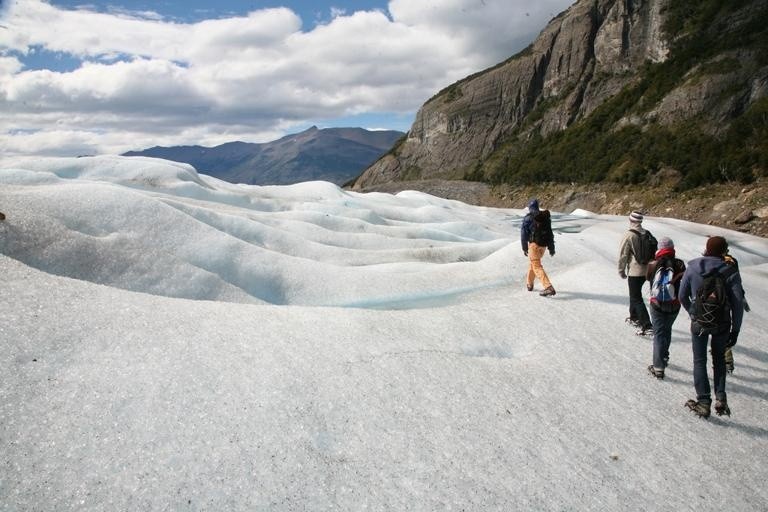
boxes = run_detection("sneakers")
[526,284,533,291]
[649,364,664,378]
[539,286,555,296]
[686,399,711,417]
[726,363,734,373]
[626,318,653,336]
[716,400,730,416]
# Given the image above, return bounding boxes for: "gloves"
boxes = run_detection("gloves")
[726,332,737,348]
[524,250,528,256]
[619,271,627,279]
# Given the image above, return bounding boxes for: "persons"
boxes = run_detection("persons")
[521,199,556,297]
[618,210,655,336]
[645,236,686,379]
[678,235,743,419]
[724,245,750,374]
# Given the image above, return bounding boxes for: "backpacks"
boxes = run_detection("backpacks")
[648,256,681,306]
[695,266,738,327]
[532,212,550,245]
[629,229,657,264]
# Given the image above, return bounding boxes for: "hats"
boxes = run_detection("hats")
[629,210,643,222]
[657,236,673,249]
[707,237,728,253]
